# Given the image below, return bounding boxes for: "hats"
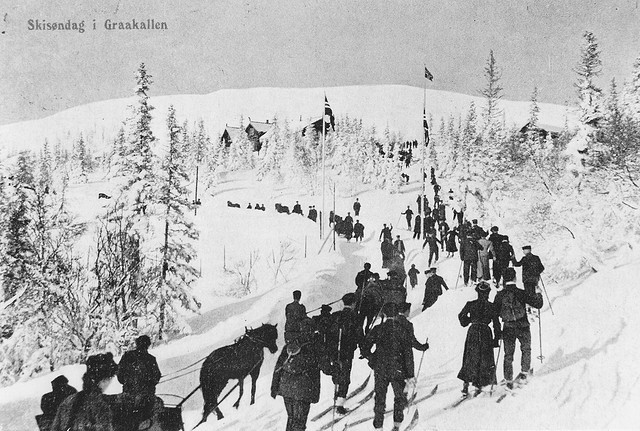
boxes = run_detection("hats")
[86,353,118,374]
[503,267,515,280]
[52,375,68,386]
[342,293,357,304]
[522,246,531,250]
[475,281,491,294]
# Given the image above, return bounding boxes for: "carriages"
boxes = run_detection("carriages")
[34,323,278,431]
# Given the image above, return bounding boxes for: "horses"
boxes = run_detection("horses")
[199,322,280,423]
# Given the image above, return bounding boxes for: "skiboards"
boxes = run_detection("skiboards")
[403,409,419,431]
[311,372,374,431]
[443,378,505,409]
[496,369,534,404]
[341,384,439,431]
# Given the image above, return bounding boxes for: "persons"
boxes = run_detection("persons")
[433,186,441,195]
[414,215,421,239]
[355,224,406,290]
[361,303,408,431]
[376,140,418,168]
[424,171,427,181]
[262,204,265,211]
[401,206,414,230]
[458,282,501,395]
[408,264,419,287]
[255,203,260,209]
[416,194,421,213]
[495,270,542,388]
[308,206,314,220]
[458,219,516,286]
[512,245,544,295]
[117,335,162,431]
[247,203,252,208]
[313,205,317,221]
[285,291,307,344]
[431,268,449,294]
[423,195,463,231]
[439,220,450,251]
[422,232,442,267]
[432,178,438,184]
[398,303,429,408]
[422,270,437,311]
[270,319,346,431]
[331,212,364,242]
[313,305,333,320]
[431,167,435,178]
[42,374,78,430]
[406,173,410,182]
[331,294,358,415]
[443,226,458,257]
[353,197,362,217]
[52,353,118,430]
[292,201,303,215]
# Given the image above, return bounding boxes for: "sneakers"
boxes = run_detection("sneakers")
[336,406,348,414]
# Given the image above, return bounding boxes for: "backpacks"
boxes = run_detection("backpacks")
[281,339,312,374]
[500,291,526,322]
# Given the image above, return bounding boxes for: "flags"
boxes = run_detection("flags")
[324,96,335,131]
[422,108,430,147]
[425,67,434,81]
